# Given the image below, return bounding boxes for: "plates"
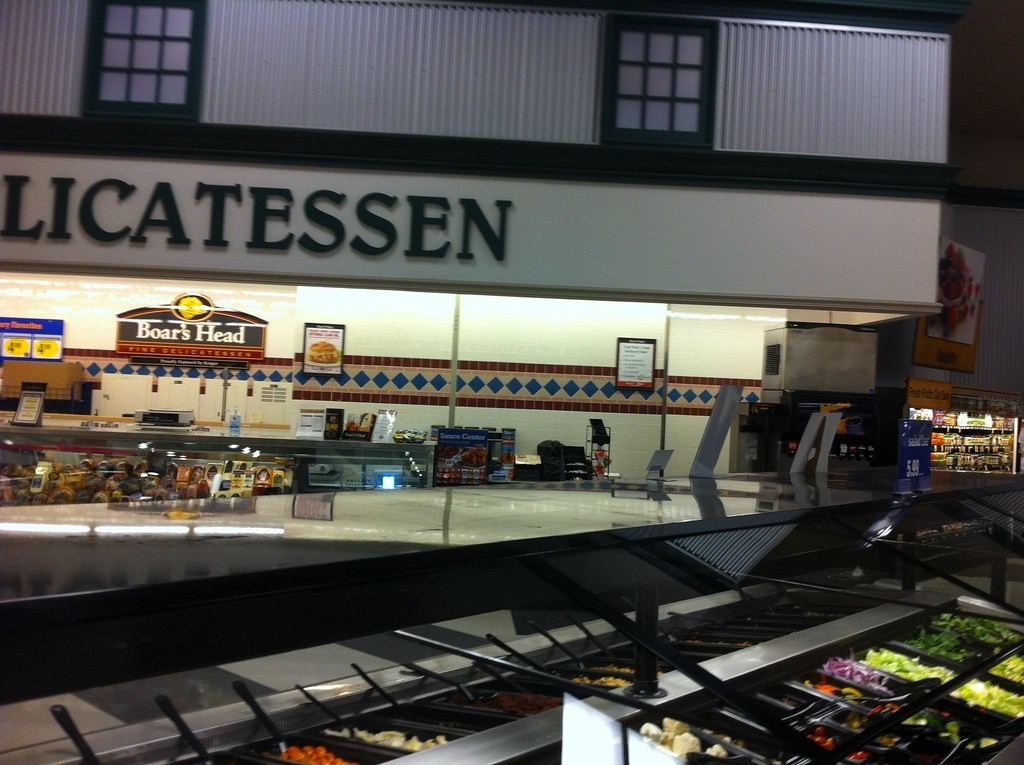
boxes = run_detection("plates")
[437,446,460,461]
[454,451,486,469]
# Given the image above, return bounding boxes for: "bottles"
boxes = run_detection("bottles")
[595,444,604,481]
[229,406,244,437]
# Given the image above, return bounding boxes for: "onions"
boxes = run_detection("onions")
[823,656,896,696]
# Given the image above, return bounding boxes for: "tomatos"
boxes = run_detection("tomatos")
[805,727,870,760]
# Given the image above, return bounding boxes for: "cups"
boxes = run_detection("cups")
[845,416,864,434]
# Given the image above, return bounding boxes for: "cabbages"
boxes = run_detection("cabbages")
[857,647,1024,719]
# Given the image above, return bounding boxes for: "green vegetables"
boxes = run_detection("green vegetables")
[899,612,1022,663]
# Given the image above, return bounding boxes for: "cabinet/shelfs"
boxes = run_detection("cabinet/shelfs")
[585,423,611,479]
[910,409,1018,474]
